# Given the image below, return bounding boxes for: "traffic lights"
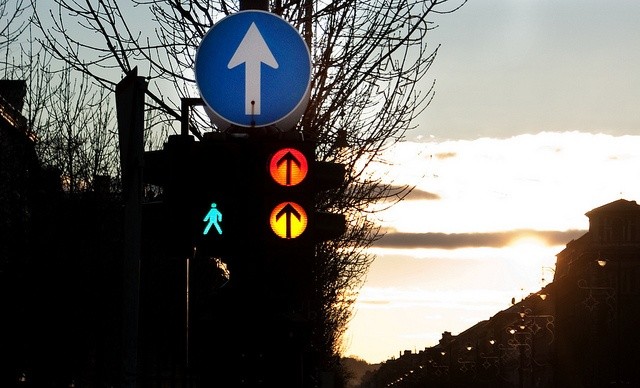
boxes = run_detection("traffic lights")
[254,139,317,252]
[313,158,348,245]
[189,142,250,256]
